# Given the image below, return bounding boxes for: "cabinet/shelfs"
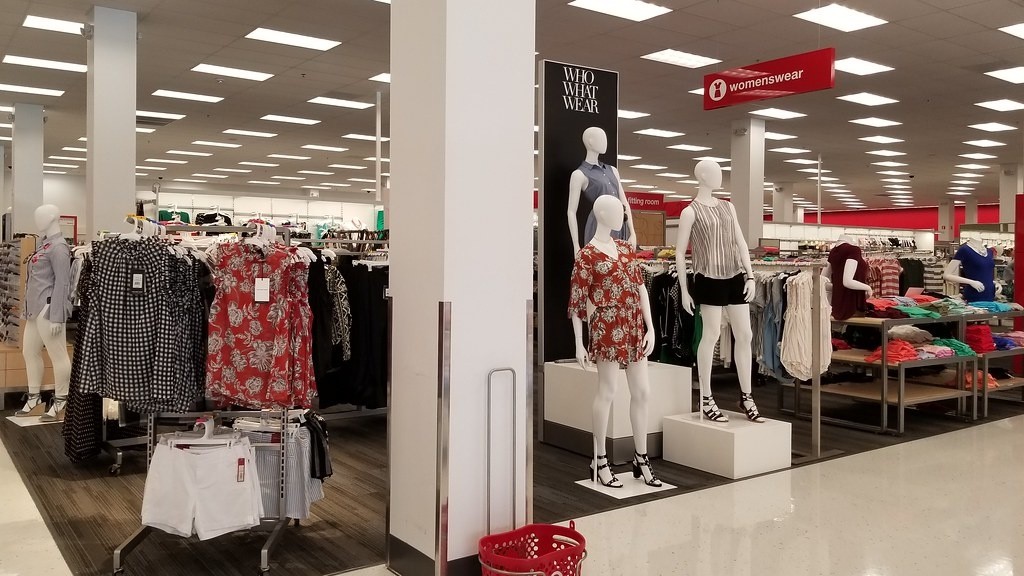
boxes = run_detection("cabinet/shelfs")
[778,310,1024,436]
[959,265,1015,289]
[0,238,73,410]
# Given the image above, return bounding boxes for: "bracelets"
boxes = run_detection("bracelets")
[747,278,754,280]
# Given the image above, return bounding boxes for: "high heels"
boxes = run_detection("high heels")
[632,450,662,487]
[38,393,68,422]
[15,392,46,417]
[698,395,729,422]
[738,391,765,422]
[589,453,623,488]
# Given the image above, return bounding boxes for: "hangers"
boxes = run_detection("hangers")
[637,259,694,278]
[232,409,310,442]
[857,233,917,250]
[166,417,240,449]
[752,259,813,284]
[864,251,936,263]
[70,201,389,271]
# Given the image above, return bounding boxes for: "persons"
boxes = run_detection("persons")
[1003,250,1015,296]
[566,126,637,261]
[675,160,767,423]
[944,237,1002,301]
[822,234,873,320]
[567,194,662,487]
[14,204,71,421]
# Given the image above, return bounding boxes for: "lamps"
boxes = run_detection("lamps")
[734,127,748,135]
[775,187,782,192]
[80,23,92,40]
